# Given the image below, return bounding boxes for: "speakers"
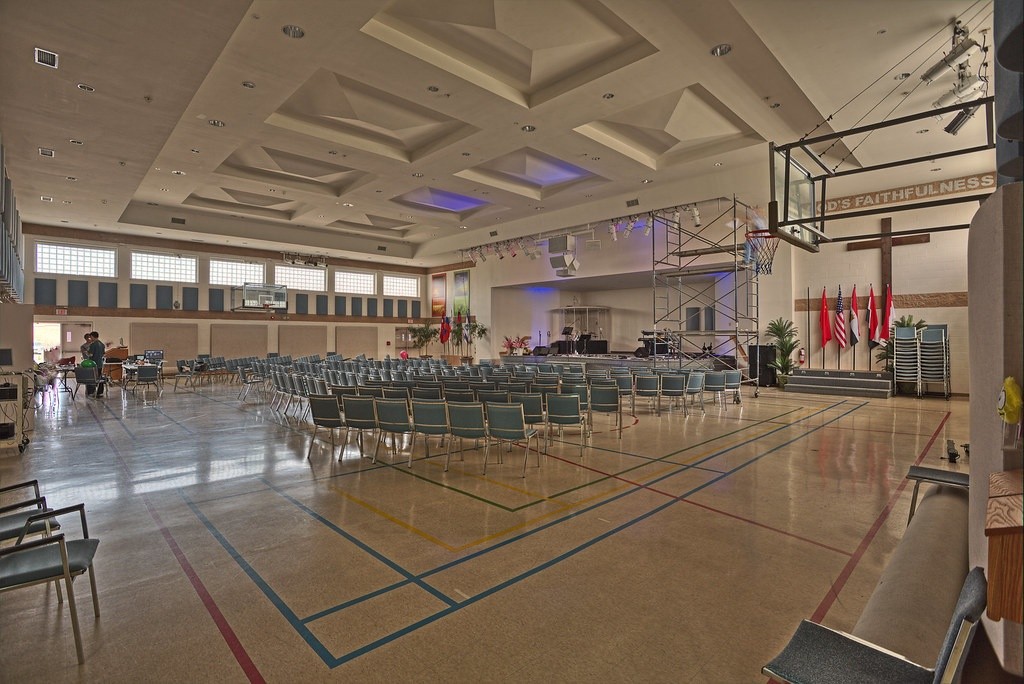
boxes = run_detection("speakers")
[550,254,573,268]
[633,347,649,358]
[549,236,575,254]
[532,346,546,356]
[749,345,782,387]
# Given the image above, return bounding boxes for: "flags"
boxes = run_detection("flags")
[866,290,881,350]
[819,289,832,348]
[879,288,897,347]
[850,288,861,347]
[440,310,471,346]
[833,287,847,348]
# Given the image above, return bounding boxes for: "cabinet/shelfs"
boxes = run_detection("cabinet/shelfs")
[0,374,30,453]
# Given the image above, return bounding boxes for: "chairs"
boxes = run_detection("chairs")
[758,560,990,684]
[890,319,952,400]
[0,345,750,671]
[903,464,969,527]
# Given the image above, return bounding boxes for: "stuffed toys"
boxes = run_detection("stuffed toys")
[997,376,1022,425]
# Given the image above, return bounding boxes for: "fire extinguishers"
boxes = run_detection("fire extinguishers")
[799,347,805,363]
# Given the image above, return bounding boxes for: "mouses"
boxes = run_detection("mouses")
[4,383,10,387]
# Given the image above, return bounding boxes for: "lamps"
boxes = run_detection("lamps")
[690,203,703,227]
[945,94,987,137]
[506,240,518,258]
[642,212,652,236]
[494,242,505,260]
[960,442,969,455]
[931,61,989,117]
[607,219,618,242]
[518,237,530,257]
[921,34,980,88]
[294,252,307,266]
[318,256,329,268]
[308,254,318,267]
[476,246,486,261]
[947,447,960,463]
[671,207,681,230]
[279,251,295,265]
[623,216,637,239]
[764,314,802,389]
[468,247,485,264]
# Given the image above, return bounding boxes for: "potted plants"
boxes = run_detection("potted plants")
[874,314,928,394]
[407,317,441,358]
[460,319,488,365]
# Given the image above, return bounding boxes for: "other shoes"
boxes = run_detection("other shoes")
[85,390,103,398]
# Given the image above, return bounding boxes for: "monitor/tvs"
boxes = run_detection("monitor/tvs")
[0,349,12,366]
[145,350,163,359]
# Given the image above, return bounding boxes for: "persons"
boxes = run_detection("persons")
[80,332,105,398]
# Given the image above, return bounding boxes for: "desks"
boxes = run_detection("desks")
[102,359,171,383]
[57,363,75,393]
[122,362,163,390]
